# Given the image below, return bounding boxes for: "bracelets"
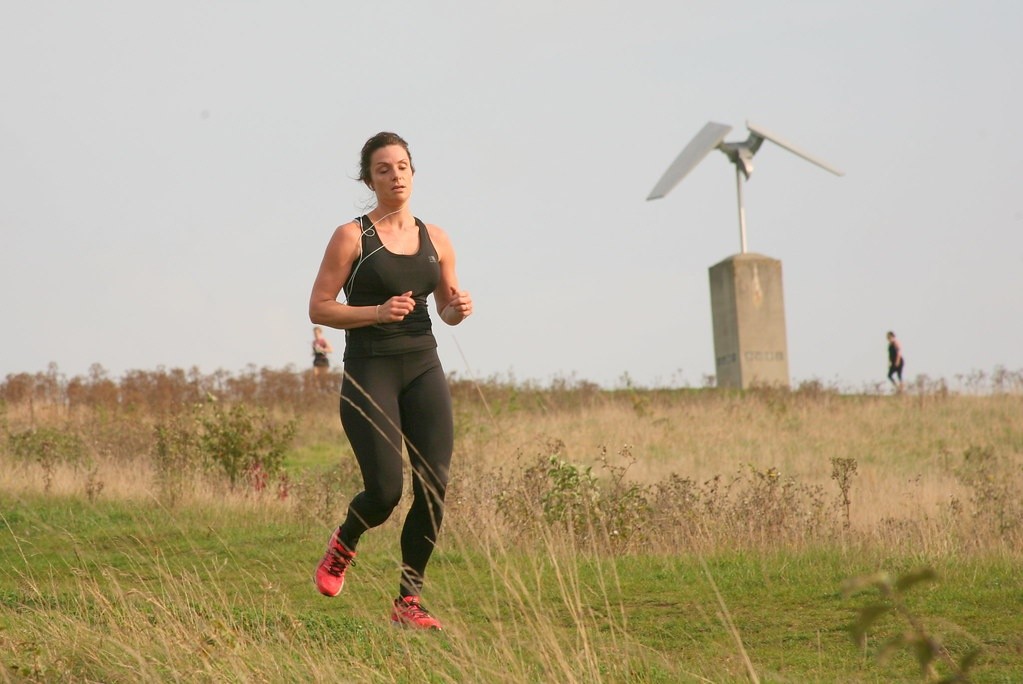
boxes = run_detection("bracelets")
[376,304,380,322]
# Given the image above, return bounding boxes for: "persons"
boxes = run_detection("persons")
[312,327,332,375]
[309,132,475,631]
[886,331,904,391]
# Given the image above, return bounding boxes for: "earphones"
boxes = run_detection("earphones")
[369,183,374,192]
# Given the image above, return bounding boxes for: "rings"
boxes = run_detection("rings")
[466,304,468,309]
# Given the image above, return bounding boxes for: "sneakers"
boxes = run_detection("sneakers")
[314,526,357,597]
[391,596,444,631]
